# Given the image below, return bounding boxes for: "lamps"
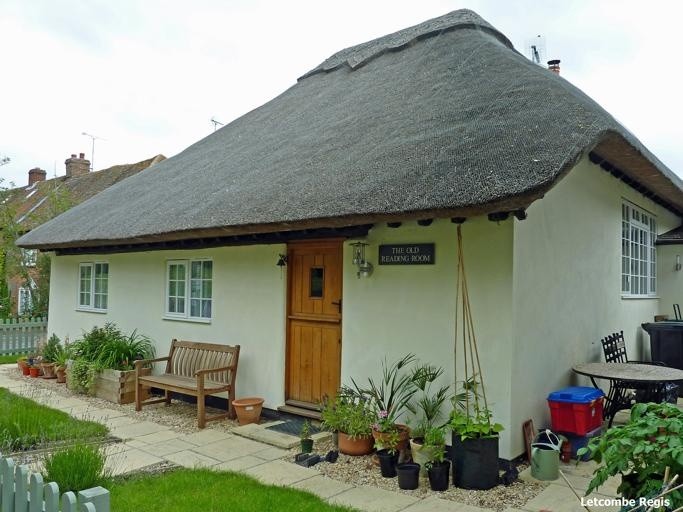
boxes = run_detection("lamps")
[348,241,374,279]
[675,254,681,270]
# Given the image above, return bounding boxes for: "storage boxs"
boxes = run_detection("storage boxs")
[546,387,606,437]
[539,427,605,463]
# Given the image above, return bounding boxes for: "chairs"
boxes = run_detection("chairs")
[601,330,679,421]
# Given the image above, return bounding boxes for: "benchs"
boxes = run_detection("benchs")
[131,337,242,428]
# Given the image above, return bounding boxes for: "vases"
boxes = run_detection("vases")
[232,397,266,426]
[30,367,39,378]
[396,463,421,490]
[34,363,44,376]
[22,366,30,377]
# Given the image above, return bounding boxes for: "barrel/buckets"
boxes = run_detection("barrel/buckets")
[531,431,568,482]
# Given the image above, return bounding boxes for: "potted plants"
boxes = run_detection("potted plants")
[400,362,456,477]
[338,351,434,456]
[334,396,373,455]
[422,442,451,492]
[320,407,338,444]
[64,321,157,405]
[443,370,505,491]
[299,419,314,452]
[372,429,400,478]
[40,333,62,380]
[53,333,74,384]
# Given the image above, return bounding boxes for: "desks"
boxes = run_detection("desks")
[571,362,683,433]
